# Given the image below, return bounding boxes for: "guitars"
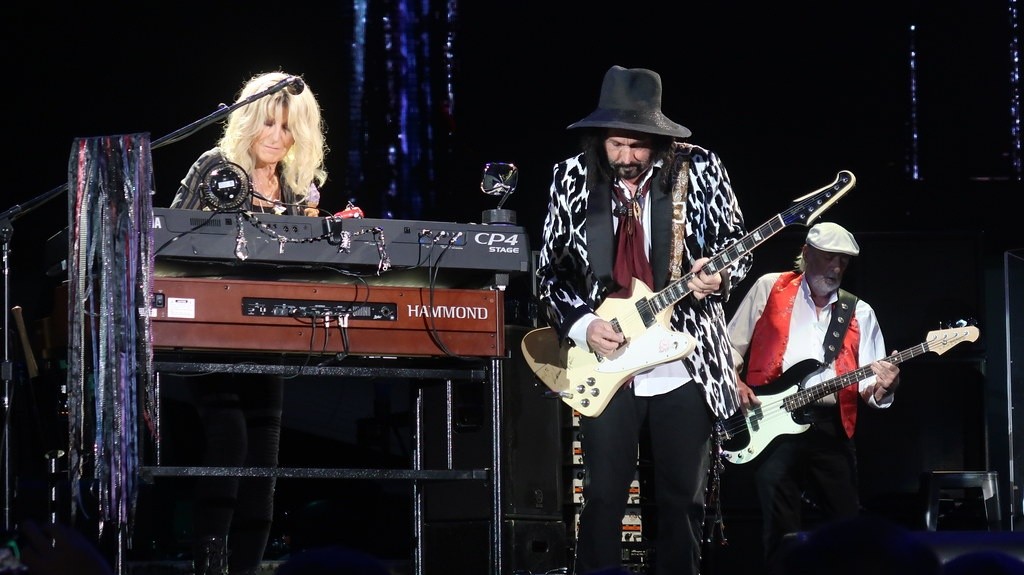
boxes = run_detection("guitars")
[520,169,856,419]
[716,318,979,467]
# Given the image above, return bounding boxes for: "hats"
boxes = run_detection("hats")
[567,65,692,138]
[806,222,860,257]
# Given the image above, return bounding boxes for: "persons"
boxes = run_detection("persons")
[168,72,327,575]
[539,65,753,574]
[724,221,902,575]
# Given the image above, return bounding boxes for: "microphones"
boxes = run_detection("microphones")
[287,77,304,95]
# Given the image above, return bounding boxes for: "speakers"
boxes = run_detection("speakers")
[502,323,579,521]
[782,528,1024,575]
[501,519,577,575]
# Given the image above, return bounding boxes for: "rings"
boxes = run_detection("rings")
[702,289,704,293]
